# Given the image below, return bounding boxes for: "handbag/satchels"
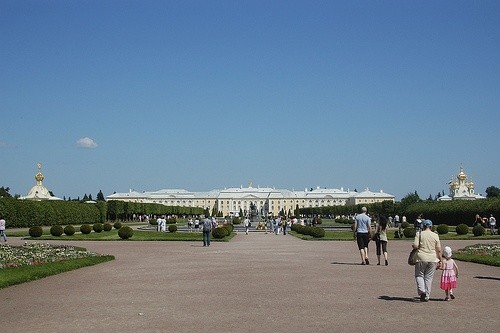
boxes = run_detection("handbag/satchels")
[408,231,421,265]
[373,233,380,241]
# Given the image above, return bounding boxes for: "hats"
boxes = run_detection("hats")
[442,246,452,258]
[423,219,433,228]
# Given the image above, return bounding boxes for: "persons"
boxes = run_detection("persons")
[473,213,496,230]
[201,215,212,247]
[353,206,372,265]
[223,216,228,225]
[0,217,7,242]
[264,217,298,235]
[244,217,250,235]
[300,217,317,227]
[372,213,388,266]
[388,213,406,228]
[211,215,218,228]
[436,246,459,301]
[156,216,167,232]
[412,219,442,302]
[188,218,200,232]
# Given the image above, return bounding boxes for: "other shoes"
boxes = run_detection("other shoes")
[424,299,428,301]
[385,260,388,266]
[445,297,449,301]
[365,258,369,264]
[362,262,365,265]
[450,293,455,299]
[420,293,427,301]
